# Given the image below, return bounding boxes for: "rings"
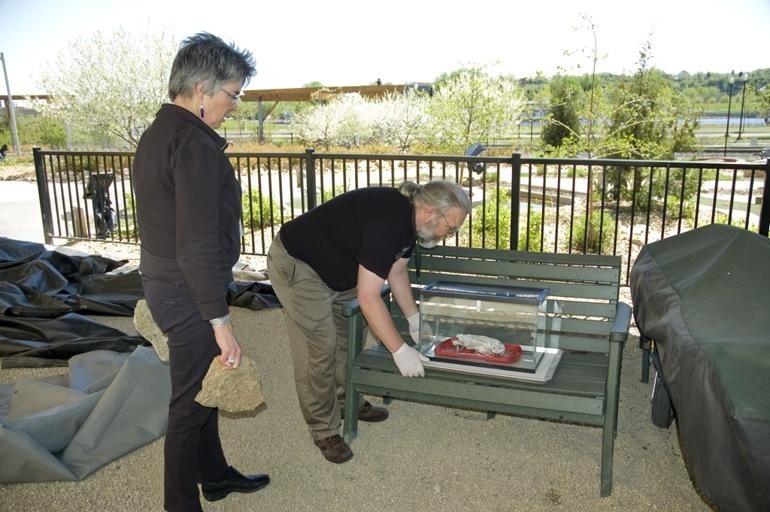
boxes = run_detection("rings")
[229,357,235,365]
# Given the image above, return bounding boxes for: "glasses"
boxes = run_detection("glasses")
[440,211,457,235]
[220,87,241,105]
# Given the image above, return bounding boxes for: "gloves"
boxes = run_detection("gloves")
[391,341,430,378]
[407,312,433,344]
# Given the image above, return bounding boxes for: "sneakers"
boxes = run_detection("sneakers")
[342,400,388,422]
[313,434,353,463]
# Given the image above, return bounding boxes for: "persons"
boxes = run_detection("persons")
[134,31,271,512]
[267,179,470,464]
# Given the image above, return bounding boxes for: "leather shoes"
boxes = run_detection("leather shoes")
[202,465,269,502]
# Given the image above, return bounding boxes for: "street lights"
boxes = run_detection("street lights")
[737,74,750,140]
[724,77,734,138]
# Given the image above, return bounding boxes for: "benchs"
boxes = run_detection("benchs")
[340,242,632,496]
